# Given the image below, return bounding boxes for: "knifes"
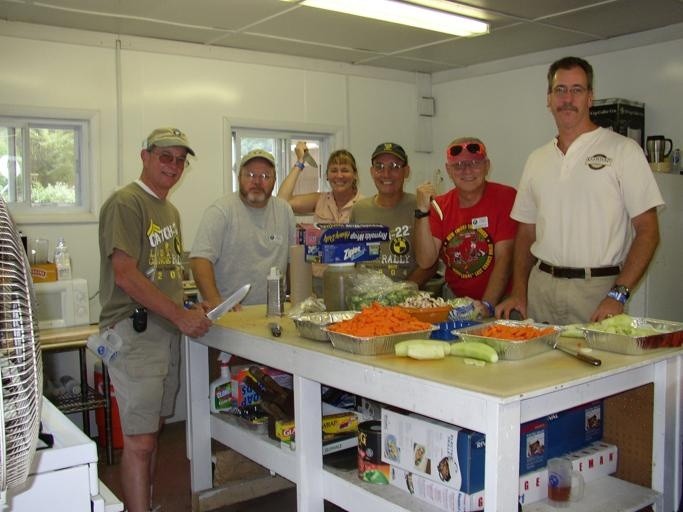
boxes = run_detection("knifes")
[269,322,283,337]
[303,150,318,170]
[427,194,444,224]
[241,365,293,421]
[556,345,601,368]
[204,284,252,323]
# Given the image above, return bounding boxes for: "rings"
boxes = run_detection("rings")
[608,313,613,317]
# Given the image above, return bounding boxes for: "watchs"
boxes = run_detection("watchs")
[610,283,630,300]
[415,209,431,219]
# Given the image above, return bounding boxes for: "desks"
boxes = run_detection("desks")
[186,297,682,509]
[3,320,114,468]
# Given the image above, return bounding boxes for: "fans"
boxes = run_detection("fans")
[0,198,48,510]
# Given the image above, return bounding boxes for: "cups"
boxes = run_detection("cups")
[84,328,124,368]
[26,238,49,266]
[645,133,672,162]
[544,457,583,511]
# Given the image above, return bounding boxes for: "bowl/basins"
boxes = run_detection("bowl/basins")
[649,162,673,174]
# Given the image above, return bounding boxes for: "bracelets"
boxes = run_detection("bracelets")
[482,300,493,312]
[607,290,626,305]
[295,161,305,170]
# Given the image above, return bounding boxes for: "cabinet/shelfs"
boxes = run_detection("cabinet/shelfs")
[2,388,125,510]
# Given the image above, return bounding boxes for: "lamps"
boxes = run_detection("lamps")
[297,0,496,38]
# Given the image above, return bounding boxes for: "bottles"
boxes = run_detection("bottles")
[673,148,680,172]
[53,238,72,280]
[39,373,82,399]
[320,263,357,316]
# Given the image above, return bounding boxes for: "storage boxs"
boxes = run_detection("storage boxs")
[582,96,643,165]
[385,438,619,508]
[380,399,608,494]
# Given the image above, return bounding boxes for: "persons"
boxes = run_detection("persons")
[349,142,438,289]
[98,128,213,512]
[495,56,665,323]
[415,139,517,315]
[189,150,298,312]
[277,141,363,228]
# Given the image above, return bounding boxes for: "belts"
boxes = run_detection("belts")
[539,263,620,278]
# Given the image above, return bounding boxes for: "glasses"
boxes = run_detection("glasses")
[447,142,485,161]
[151,151,189,169]
[452,160,482,170]
[373,162,402,173]
[554,87,584,97]
[245,170,270,181]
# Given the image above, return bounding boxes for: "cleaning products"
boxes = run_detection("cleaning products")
[210,352,239,414]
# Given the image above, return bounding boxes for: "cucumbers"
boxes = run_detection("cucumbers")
[450,341,498,363]
[408,344,444,360]
[394,339,450,357]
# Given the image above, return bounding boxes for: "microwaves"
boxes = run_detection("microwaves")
[25,279,90,330]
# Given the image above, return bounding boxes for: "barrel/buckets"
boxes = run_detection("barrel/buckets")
[357,420,391,485]
[93,360,166,450]
[323,262,357,312]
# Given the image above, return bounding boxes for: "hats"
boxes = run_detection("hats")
[240,149,275,167]
[147,127,195,157]
[371,143,406,161]
